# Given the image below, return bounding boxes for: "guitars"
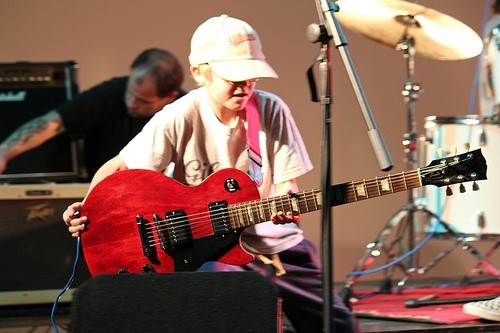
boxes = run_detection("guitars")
[71,147,489,277]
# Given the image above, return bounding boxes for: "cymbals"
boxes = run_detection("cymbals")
[325,0,484,63]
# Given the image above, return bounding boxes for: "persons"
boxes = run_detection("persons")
[461,294,500,322]
[61,14,353,333]
[0,47,187,183]
[478,0,500,122]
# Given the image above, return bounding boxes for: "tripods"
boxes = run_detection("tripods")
[337,29,493,305]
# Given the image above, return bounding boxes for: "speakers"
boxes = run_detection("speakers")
[69,271,279,333]
[0,61,91,185]
[0,182,92,316]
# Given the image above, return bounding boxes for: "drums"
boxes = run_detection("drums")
[476,13,500,123]
[420,113,500,238]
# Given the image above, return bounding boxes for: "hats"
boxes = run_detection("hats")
[188,14,279,82]
